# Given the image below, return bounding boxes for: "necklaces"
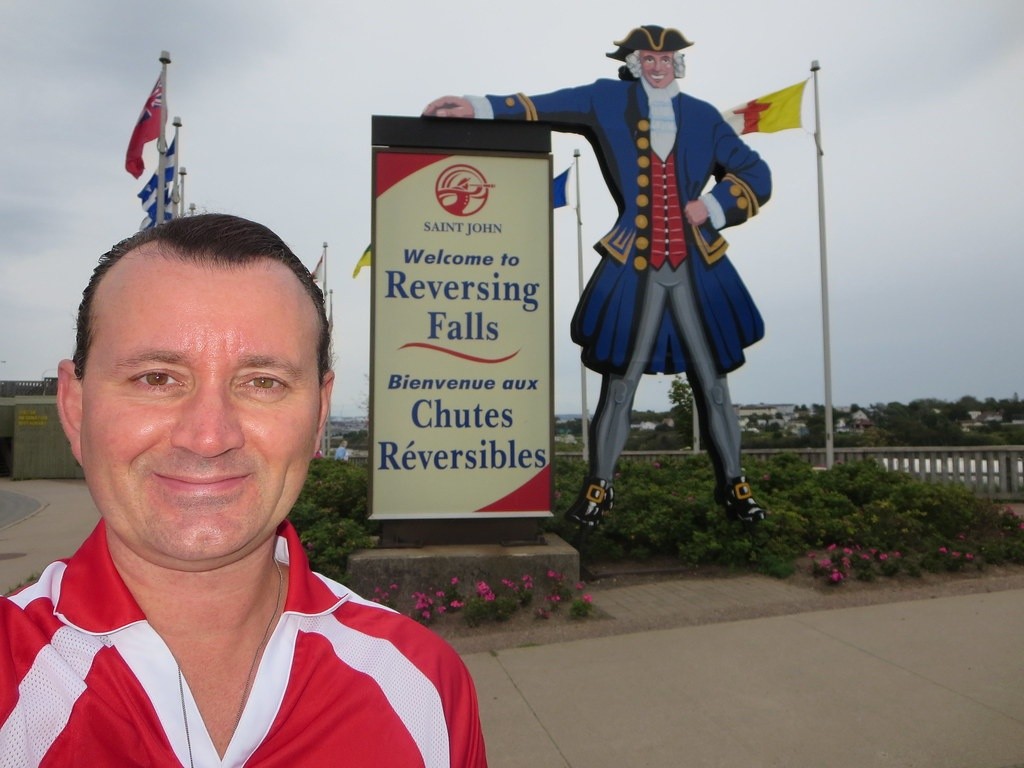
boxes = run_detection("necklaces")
[149,556,284,768]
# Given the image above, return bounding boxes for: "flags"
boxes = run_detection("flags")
[125,75,176,231]
[311,253,323,284]
[352,243,371,280]
[719,76,807,137]
[553,166,573,209]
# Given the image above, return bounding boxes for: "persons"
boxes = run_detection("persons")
[0,212,487,767]
[334,440,348,460]
